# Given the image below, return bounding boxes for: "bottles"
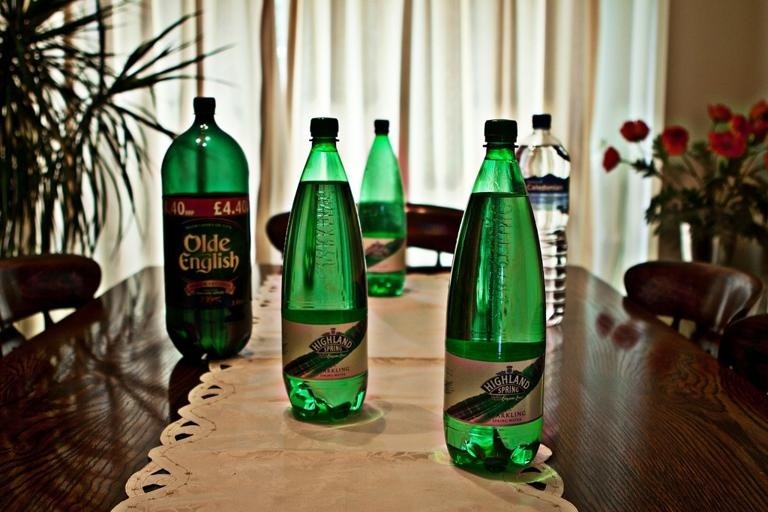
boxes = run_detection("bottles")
[445,120,546,470]
[514,113,570,327]
[282,118,368,424]
[161,97,252,355]
[358,120,407,297]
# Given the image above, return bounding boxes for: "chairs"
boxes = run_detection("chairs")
[719,312,768,392]
[1,253,103,326]
[623,258,763,346]
[267,203,464,276]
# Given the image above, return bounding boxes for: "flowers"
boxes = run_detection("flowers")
[600,98,768,254]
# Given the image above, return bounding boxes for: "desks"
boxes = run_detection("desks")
[0,262,768,511]
